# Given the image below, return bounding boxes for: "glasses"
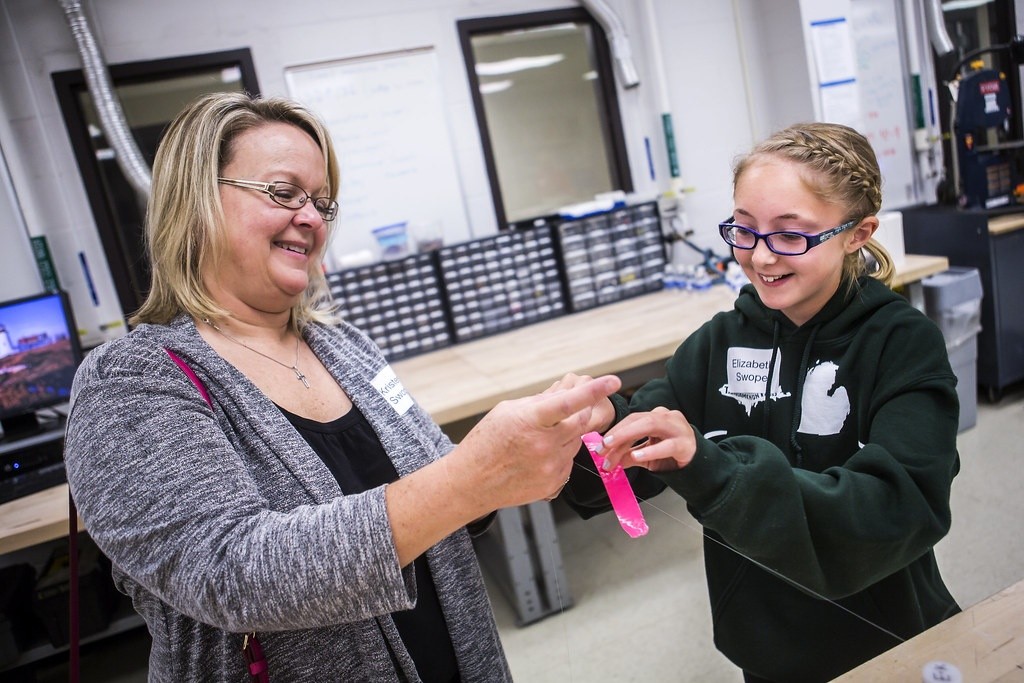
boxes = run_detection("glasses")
[217,177,339,222]
[719,216,865,257]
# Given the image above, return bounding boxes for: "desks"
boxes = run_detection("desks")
[0,486,88,575]
[389,254,946,622]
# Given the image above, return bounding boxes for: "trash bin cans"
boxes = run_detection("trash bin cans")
[921,262,985,436]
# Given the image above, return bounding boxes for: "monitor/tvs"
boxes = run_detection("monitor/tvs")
[0,290,84,445]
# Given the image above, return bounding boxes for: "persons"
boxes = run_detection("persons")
[64,92,622,683]
[543,122,961,683]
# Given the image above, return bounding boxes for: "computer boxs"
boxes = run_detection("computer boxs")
[0,407,68,506]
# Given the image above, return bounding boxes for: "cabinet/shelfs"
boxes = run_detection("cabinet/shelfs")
[311,200,668,358]
[902,201,1024,408]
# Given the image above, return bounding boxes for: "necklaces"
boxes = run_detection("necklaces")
[198,317,311,388]
